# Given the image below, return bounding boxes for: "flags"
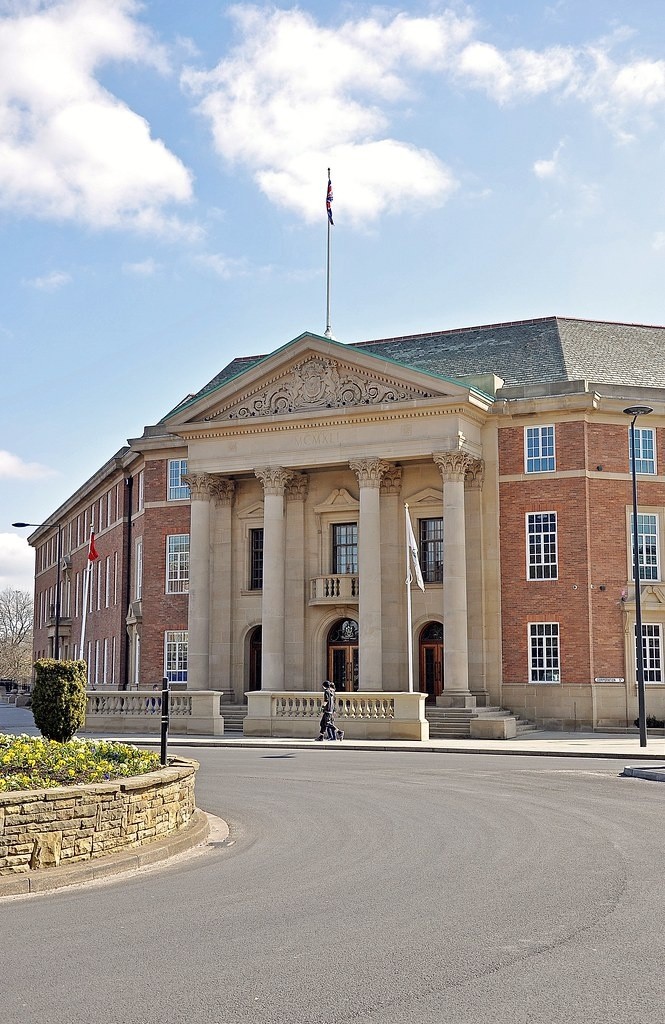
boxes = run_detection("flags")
[406,510,426,594]
[326,178,335,226]
[88,531,99,561]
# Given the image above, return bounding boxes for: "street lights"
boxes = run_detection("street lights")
[626,406,652,748]
[13,522,62,661]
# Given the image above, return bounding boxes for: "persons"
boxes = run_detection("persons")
[145,684,161,714]
[315,681,344,742]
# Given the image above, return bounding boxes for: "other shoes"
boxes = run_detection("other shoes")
[328,738,336,741]
[325,736,332,739]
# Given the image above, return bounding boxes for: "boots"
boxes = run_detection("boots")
[315,734,324,741]
[337,730,345,741]
